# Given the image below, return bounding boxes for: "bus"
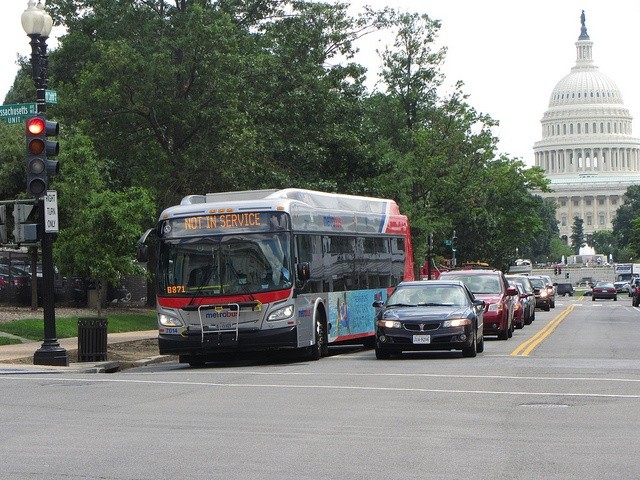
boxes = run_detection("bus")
[136,188,415,366]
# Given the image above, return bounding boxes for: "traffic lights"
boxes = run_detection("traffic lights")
[45,120,60,177]
[25,115,47,196]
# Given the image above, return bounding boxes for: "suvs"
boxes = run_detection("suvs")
[553,283,574,298]
[613,274,640,292]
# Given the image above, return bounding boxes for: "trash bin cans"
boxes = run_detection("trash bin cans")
[77,317,108,362]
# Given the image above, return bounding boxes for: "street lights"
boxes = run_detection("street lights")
[21,0,70,367]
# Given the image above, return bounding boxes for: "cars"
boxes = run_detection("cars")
[584,288,593,296]
[629,277,640,296]
[507,282,527,329]
[25,264,63,296]
[0,263,42,306]
[516,283,530,326]
[372,280,484,361]
[441,270,517,341]
[632,283,640,307]
[505,275,535,324]
[593,282,617,302]
[529,277,550,312]
[536,275,555,308]
